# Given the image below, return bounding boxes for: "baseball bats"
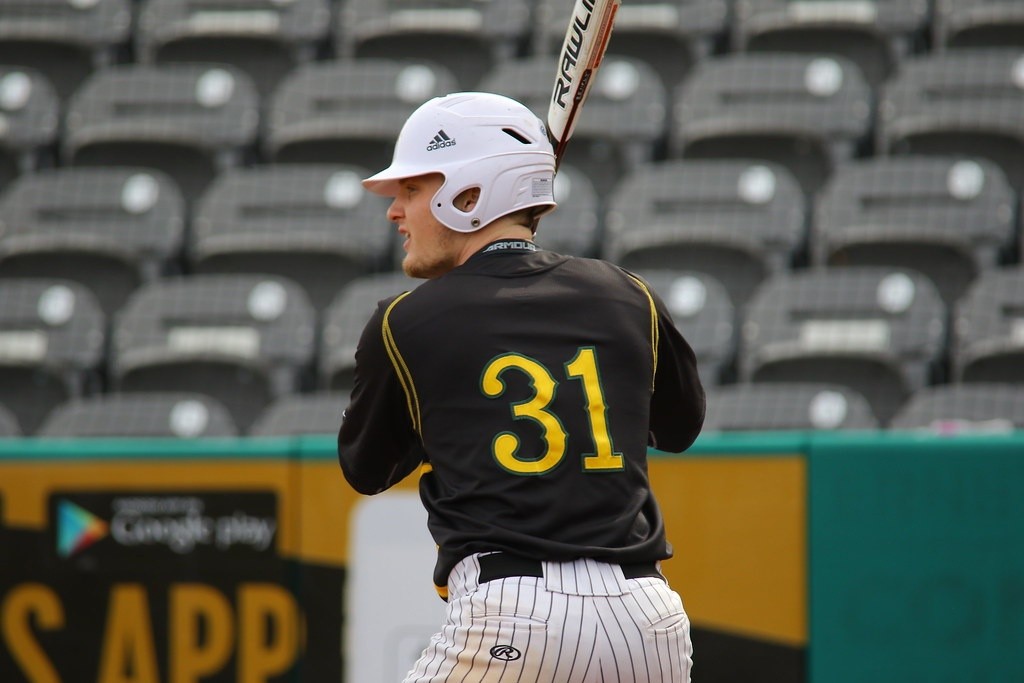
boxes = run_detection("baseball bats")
[531,0,621,244]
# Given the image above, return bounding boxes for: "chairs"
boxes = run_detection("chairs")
[0,0,1024,441]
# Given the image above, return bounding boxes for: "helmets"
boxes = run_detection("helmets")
[360,89,557,233]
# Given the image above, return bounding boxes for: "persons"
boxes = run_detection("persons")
[335,91,709,683]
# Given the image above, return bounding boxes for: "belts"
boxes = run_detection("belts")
[477,542,665,587]
